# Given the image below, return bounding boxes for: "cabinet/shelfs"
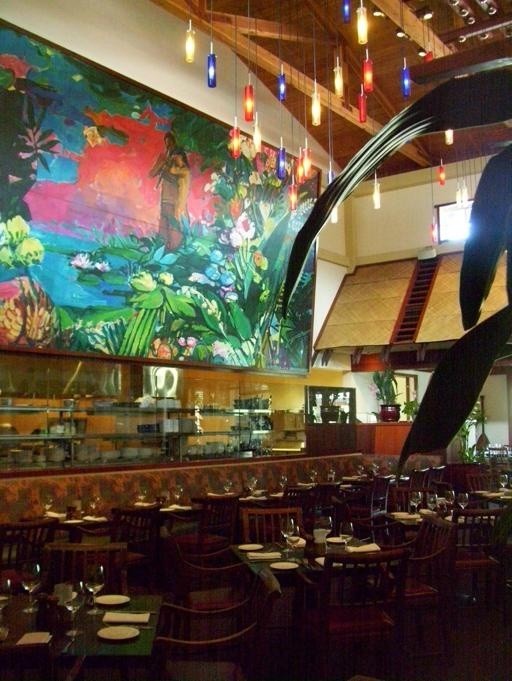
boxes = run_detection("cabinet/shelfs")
[0,406,275,473]
[306,422,413,455]
[0,450,363,523]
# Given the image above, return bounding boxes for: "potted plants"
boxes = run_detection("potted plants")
[319,388,340,424]
[373,369,401,422]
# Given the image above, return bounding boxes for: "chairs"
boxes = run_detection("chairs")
[0,518,58,570]
[39,544,127,598]
[0,643,87,681]
[79,503,160,594]
[349,477,390,543]
[429,466,446,491]
[171,495,238,576]
[451,508,505,615]
[154,568,282,681]
[409,468,430,490]
[291,549,412,681]
[378,514,458,667]
[431,480,452,498]
[282,484,317,536]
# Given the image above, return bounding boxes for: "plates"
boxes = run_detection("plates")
[269,561,299,570]
[238,543,264,551]
[96,625,141,641]
[326,537,346,543]
[94,594,131,606]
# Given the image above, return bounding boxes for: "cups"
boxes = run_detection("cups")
[19,559,43,614]
[457,493,469,510]
[62,579,87,638]
[426,494,438,515]
[278,474,289,495]
[84,565,107,615]
[411,491,422,514]
[245,476,258,497]
[499,474,509,494]
[314,515,333,555]
[444,490,456,516]
[38,487,55,520]
[279,518,295,554]
[338,521,355,555]
[171,483,184,506]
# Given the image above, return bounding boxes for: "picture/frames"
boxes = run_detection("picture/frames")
[0,19,323,377]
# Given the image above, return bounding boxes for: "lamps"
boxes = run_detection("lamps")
[289,175,297,210]
[363,49,373,93]
[302,137,311,181]
[425,51,432,63]
[311,79,321,126]
[439,157,445,186]
[244,72,253,122]
[297,146,304,185]
[401,57,410,99]
[208,42,216,87]
[333,57,343,97]
[445,129,453,145]
[277,136,286,180]
[253,112,262,153]
[185,19,196,63]
[343,1,351,24]
[332,208,338,223]
[430,217,438,244]
[359,83,367,123]
[456,182,462,209]
[278,64,285,102]
[462,181,468,208]
[373,171,380,210]
[232,117,240,158]
[327,161,335,185]
[356,0,368,44]
